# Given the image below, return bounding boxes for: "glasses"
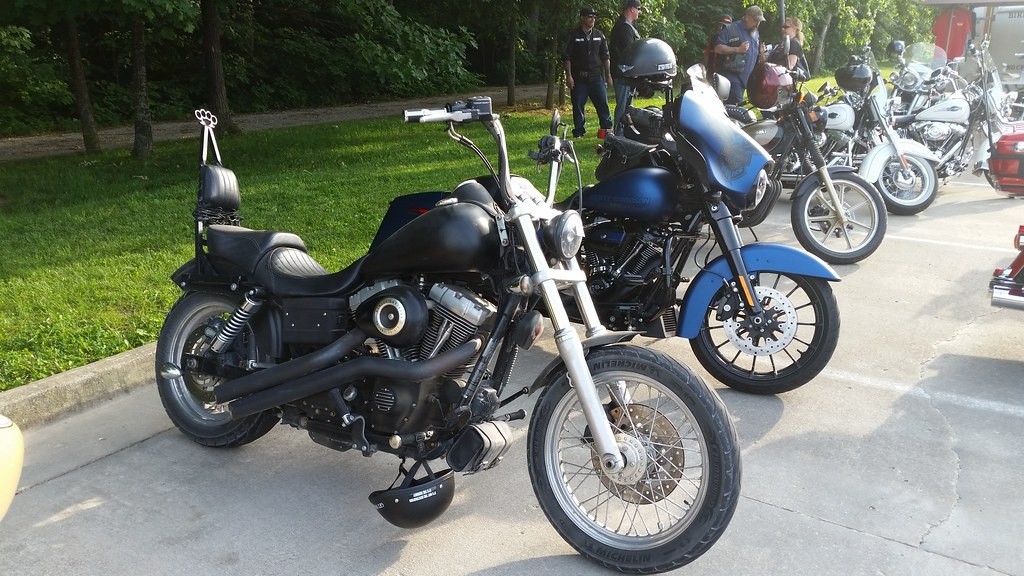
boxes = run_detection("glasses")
[635,7,641,10]
[589,16,596,19]
[782,26,790,28]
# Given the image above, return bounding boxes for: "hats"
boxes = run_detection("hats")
[580,8,600,16]
[718,15,732,26]
[746,6,766,22]
[627,0,640,7]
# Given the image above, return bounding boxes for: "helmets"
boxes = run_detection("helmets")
[835,64,872,91]
[747,63,794,111]
[886,40,906,53]
[616,38,677,78]
[368,468,455,528]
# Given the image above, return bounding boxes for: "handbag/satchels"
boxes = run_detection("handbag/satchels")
[595,133,659,182]
[786,40,811,82]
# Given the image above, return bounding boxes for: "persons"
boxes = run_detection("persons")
[562,6,613,138]
[704,5,805,107]
[609,0,643,136]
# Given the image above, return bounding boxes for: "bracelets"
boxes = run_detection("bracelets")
[567,73,572,76]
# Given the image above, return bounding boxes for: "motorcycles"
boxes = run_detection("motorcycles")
[372,61,846,397]
[154,92,742,576]
[621,12,1024,266]
[983,129,1023,312]
